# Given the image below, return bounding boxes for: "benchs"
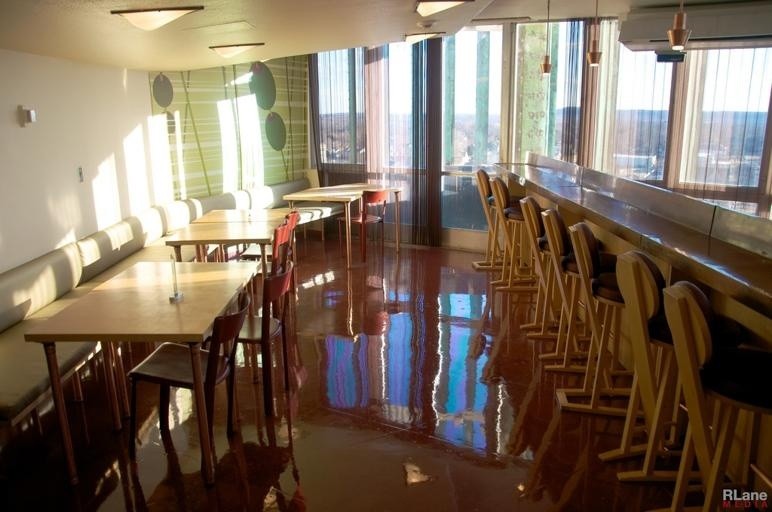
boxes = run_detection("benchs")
[1,177,312,451]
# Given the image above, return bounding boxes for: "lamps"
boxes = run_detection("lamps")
[585,0,603,67]
[414,0,475,18]
[667,0,692,50]
[539,0,553,74]
[110,5,205,32]
[208,42,264,59]
[404,32,447,47]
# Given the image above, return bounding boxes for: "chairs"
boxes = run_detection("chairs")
[22,183,403,486]
[468,166,771,512]
